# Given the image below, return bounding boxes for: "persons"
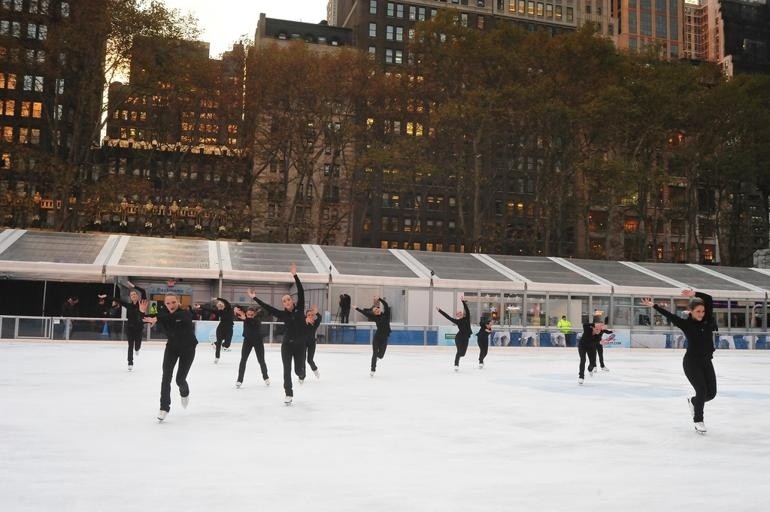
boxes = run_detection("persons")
[477,316,494,366]
[593,323,617,372]
[577,323,606,385]
[557,314,572,345]
[59,294,351,345]
[639,288,717,435]
[104,280,146,368]
[193,297,234,364]
[353,294,391,373]
[137,291,197,422]
[248,260,308,403]
[230,306,274,389]
[435,296,472,371]
[300,307,322,378]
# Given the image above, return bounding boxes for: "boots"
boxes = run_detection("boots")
[182,397,189,407]
[687,397,707,433]
[235,380,241,389]
[370,370,376,380]
[265,378,271,386]
[157,409,169,420]
[478,363,485,372]
[284,369,320,404]
[577,366,609,384]
[127,365,133,371]
[211,340,230,364]
[135,349,141,357]
